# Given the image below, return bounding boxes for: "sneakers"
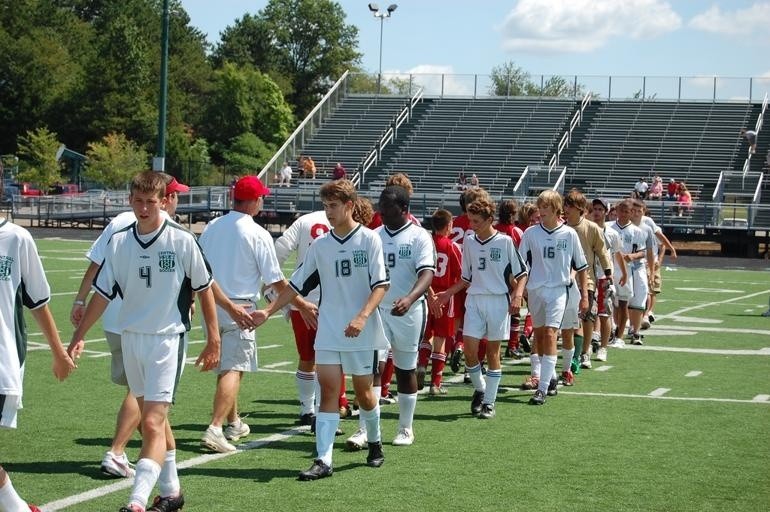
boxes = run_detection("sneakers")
[471,390,495,418]
[101,451,184,512]
[505,334,531,359]
[299,459,332,481]
[346,427,415,467]
[225,422,250,441]
[522,311,654,405]
[418,348,486,395]
[201,428,236,453]
[300,393,397,435]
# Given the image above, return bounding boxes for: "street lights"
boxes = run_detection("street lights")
[368,3,399,92]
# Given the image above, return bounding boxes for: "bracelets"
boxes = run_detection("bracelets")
[72,299,88,306]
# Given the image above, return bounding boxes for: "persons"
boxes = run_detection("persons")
[68,170,260,478]
[278,156,344,188]
[227,174,241,190]
[195,176,321,453]
[259,173,700,480]
[65,169,223,512]
[740,127,758,154]
[0,159,76,511]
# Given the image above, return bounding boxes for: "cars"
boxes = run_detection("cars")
[1,180,233,226]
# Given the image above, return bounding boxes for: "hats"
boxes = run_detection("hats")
[167,177,190,194]
[235,176,269,200]
